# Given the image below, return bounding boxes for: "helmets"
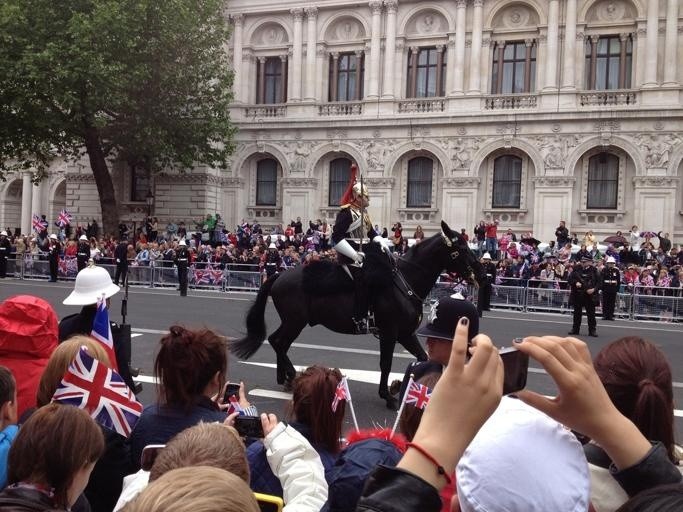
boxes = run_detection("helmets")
[79,234,87,241]
[61,265,121,309]
[49,233,57,240]
[352,182,369,198]
[0,230,8,237]
[413,290,481,348]
[606,256,616,264]
[482,253,491,261]
[267,243,276,250]
[178,240,187,246]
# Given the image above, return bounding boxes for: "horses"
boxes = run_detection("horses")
[224,220,488,411]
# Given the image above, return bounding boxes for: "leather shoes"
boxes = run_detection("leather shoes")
[589,330,598,338]
[569,330,580,335]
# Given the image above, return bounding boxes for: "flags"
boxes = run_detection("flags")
[54,209,72,229]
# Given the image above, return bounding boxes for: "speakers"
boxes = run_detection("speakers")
[15,227,22,236]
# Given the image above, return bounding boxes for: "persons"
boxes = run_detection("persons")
[0,214,683,337]
[331,164,394,330]
[0,265,682,512]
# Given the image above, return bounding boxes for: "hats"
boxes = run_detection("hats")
[580,255,591,263]
[452,394,594,510]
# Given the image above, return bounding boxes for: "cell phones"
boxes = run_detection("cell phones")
[222,382,240,404]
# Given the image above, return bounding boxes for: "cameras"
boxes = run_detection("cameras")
[498,346,529,396]
[234,416,264,438]
[140,444,167,471]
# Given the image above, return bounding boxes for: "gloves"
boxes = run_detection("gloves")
[372,234,392,255]
[335,240,366,264]
